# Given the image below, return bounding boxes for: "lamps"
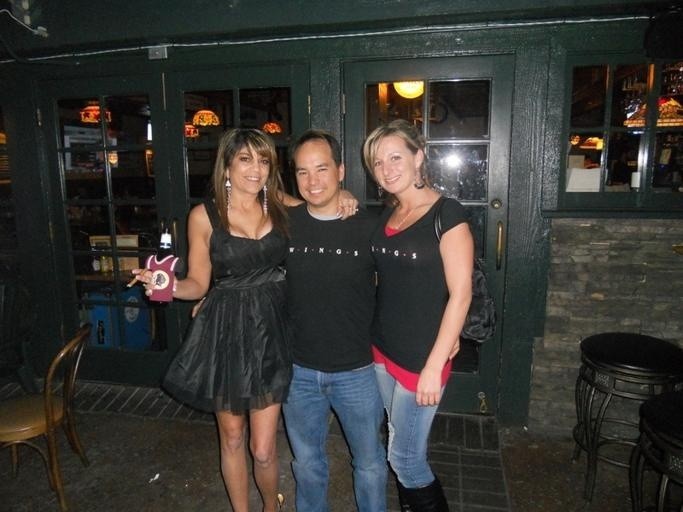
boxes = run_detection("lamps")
[391,81,426,99]
[262,120,283,136]
[190,108,220,130]
[79,105,112,126]
[185,122,199,138]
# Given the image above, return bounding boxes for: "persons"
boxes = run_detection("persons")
[191,129,460,512]
[131,129,360,512]
[362,118,475,512]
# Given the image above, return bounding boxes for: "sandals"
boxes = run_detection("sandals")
[277,493,286,512]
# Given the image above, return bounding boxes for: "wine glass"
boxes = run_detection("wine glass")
[615,65,681,103]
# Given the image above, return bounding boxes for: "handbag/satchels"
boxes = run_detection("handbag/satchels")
[434,197,499,344]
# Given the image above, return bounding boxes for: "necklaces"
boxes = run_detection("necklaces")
[394,196,424,230]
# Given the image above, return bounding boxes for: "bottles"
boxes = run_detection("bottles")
[616,135,675,191]
[144,227,178,302]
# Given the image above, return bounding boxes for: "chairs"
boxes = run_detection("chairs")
[0,322,94,512]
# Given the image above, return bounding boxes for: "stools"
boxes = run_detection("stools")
[627,392,683,511]
[572,331,683,504]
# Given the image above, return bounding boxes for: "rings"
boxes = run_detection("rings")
[353,208,358,211]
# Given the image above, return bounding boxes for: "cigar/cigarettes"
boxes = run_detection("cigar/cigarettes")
[126,267,147,287]
